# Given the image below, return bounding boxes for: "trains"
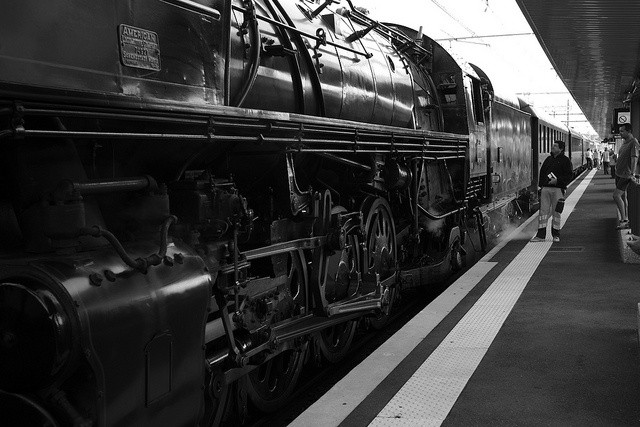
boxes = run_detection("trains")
[2,0,594,427]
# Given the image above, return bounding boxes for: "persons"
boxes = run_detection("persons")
[530,140,574,241]
[612,123,639,228]
[583,147,613,174]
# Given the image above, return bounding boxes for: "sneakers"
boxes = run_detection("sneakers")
[553,235,560,242]
[529,235,546,241]
